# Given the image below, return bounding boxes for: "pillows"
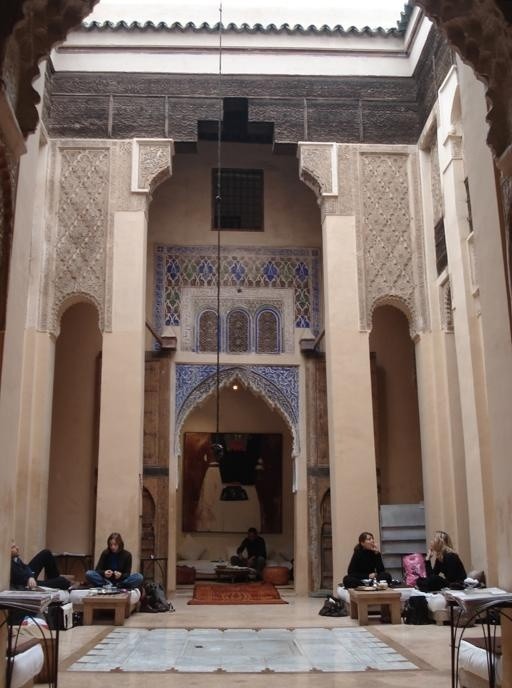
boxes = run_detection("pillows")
[465,567,485,587]
[224,544,248,558]
[178,532,206,560]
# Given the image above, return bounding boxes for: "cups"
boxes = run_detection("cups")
[90,588,98,596]
[97,586,118,593]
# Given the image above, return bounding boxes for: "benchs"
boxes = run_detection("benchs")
[0,581,140,617]
[337,575,490,626]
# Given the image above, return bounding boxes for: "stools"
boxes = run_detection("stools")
[261,566,290,586]
[4,631,46,688]
[175,563,197,585]
[457,635,512,688]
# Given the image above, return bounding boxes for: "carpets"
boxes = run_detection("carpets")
[186,582,289,604]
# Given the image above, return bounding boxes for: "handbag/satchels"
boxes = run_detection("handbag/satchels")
[400,552,426,587]
[318,593,350,617]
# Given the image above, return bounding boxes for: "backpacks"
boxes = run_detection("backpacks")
[400,593,434,625]
[138,581,176,614]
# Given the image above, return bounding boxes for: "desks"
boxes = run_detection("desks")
[347,587,402,625]
[140,554,169,590]
[0,601,62,687]
[81,592,133,625]
[445,599,512,687]
[214,568,251,582]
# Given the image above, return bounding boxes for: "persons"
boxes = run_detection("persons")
[343,531,392,589]
[415,530,467,592]
[11,539,73,589]
[231,527,267,579]
[85,532,144,591]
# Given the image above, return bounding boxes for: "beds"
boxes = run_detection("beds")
[177,557,293,579]
